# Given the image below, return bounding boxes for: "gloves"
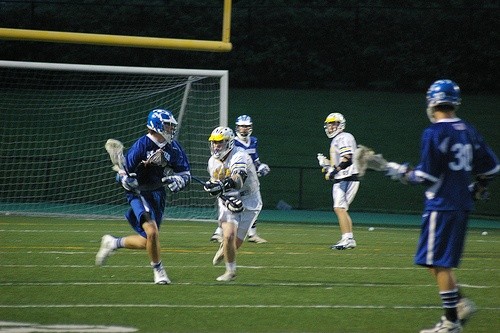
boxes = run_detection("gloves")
[257,163,271,177]
[325,165,338,180]
[223,196,245,213]
[204,177,231,197]
[162,175,188,192]
[468,182,488,201]
[120,172,139,192]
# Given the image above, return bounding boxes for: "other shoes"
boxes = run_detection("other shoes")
[216,270,236,281]
[385,162,409,182]
[95,234,116,267]
[330,238,356,250]
[211,226,224,242]
[436,297,477,327]
[213,241,225,265]
[418,315,464,333]
[248,233,268,244]
[153,267,170,285]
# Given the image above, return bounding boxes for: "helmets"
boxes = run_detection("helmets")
[235,114,253,141]
[324,113,346,138]
[425,80,462,124]
[208,126,234,161]
[146,109,178,145]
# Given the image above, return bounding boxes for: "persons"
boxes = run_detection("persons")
[95,109,192,285]
[385,79,500,333]
[204,126,263,281]
[210,115,271,243]
[317,113,361,250]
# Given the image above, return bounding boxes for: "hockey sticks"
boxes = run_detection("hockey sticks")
[190,174,245,213]
[353,143,491,202]
[105,138,153,227]
[317,152,338,179]
